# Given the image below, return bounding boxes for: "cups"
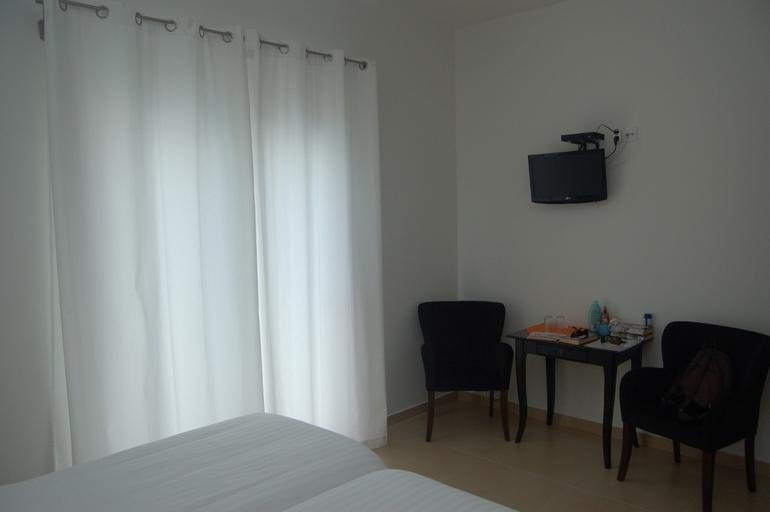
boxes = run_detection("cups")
[542,314,567,332]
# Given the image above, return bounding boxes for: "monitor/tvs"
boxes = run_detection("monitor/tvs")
[527,148,608,204]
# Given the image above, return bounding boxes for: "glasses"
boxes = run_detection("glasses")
[601,336,626,345]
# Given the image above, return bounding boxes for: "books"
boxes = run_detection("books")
[560,334,597,345]
[611,323,654,343]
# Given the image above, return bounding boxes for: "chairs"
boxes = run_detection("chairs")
[617,321,769,512]
[417,300,514,443]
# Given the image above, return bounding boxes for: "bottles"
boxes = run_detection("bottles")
[642,313,652,327]
[588,299,610,336]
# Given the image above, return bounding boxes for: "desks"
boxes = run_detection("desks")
[504,322,653,469]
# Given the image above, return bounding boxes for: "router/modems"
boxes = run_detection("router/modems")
[561,132,605,143]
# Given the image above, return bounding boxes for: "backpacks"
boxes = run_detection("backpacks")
[661,334,733,419]
